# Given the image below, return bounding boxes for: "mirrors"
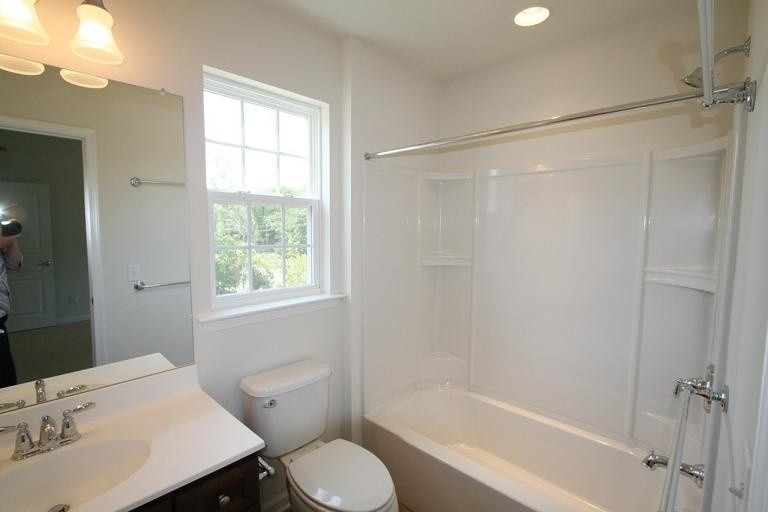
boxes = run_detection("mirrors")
[0,115,108,388]
[0,54,195,414]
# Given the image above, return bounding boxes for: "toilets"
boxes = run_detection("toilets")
[239,357,400,512]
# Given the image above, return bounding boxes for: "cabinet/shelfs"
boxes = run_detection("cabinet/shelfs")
[129,451,261,512]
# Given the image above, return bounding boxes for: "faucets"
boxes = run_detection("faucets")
[35,379,47,404]
[38,415,57,453]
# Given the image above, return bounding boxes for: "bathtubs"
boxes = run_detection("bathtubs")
[363,381,703,512]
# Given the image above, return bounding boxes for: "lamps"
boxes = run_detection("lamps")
[60,68,109,89]
[69,1,123,65]
[0,54,45,76]
[0,0,49,47]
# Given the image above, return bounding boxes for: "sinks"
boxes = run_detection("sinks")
[0,375,113,410]
[0,438,152,512]
